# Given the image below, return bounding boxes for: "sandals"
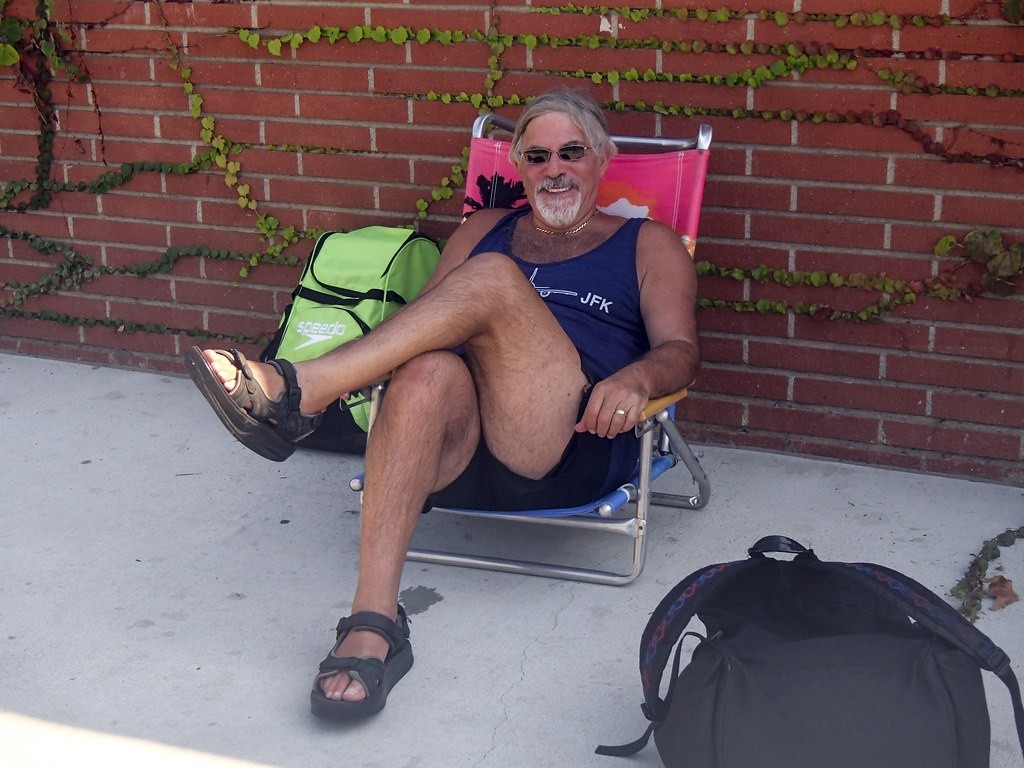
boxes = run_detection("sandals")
[309,604,415,720]
[183,344,325,459]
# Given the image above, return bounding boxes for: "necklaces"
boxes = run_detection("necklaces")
[533,211,600,235]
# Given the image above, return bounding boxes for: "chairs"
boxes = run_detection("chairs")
[349,113,714,585]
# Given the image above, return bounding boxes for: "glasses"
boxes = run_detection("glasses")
[520,143,592,165]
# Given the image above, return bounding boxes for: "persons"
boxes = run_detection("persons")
[188,93,701,719]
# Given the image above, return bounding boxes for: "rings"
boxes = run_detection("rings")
[616,410,626,416]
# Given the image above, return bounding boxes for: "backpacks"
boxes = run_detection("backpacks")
[259,224,444,455]
[596,536,1024,768]
